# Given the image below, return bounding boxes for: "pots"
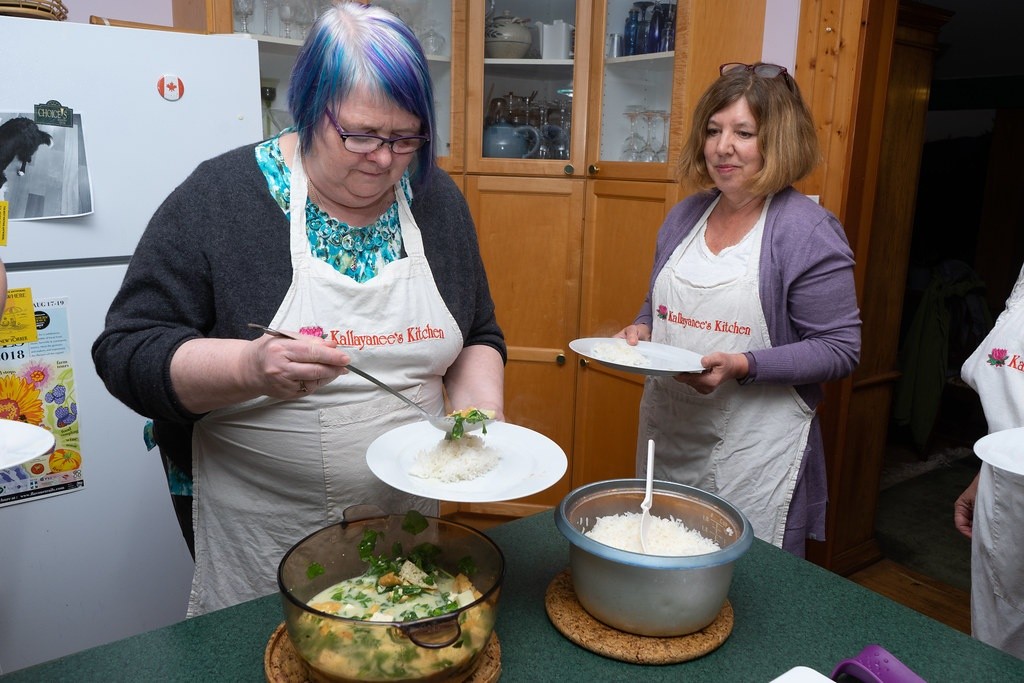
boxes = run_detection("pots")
[553,477,755,637]
[276,504,506,682]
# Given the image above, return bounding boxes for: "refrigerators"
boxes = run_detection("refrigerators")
[0,13,264,675]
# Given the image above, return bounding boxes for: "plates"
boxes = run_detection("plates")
[568,337,706,377]
[973,426,1024,476]
[0,419,56,471]
[366,421,568,503]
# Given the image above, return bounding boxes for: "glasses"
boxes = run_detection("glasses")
[322,106,432,155]
[719,62,794,93]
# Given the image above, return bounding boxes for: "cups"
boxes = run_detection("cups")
[607,33,623,57]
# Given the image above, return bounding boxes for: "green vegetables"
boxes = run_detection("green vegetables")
[444,409,489,439]
[300,510,477,681]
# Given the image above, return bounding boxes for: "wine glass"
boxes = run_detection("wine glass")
[621,109,668,162]
[231,0,321,44]
[416,18,446,56]
[506,95,572,161]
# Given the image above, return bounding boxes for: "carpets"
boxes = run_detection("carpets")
[873,455,983,596]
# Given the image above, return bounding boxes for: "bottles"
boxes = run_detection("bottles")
[624,4,676,54]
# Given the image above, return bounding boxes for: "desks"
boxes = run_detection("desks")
[1,507,1023,683]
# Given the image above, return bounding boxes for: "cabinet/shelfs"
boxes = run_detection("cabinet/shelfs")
[173,0,767,531]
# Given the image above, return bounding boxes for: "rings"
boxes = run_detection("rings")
[300,381,308,392]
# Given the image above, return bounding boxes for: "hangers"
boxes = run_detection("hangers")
[943,255,983,294]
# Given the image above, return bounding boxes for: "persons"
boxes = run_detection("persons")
[92,0,508,619]
[953,260,1024,661]
[609,61,861,562]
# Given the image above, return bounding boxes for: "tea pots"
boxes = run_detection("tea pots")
[484,122,540,159]
[535,19,575,59]
[485,9,532,59]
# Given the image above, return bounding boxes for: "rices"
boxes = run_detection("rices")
[589,341,652,367]
[407,431,503,483]
[578,512,734,556]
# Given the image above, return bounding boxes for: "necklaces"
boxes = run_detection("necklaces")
[308,181,384,220]
